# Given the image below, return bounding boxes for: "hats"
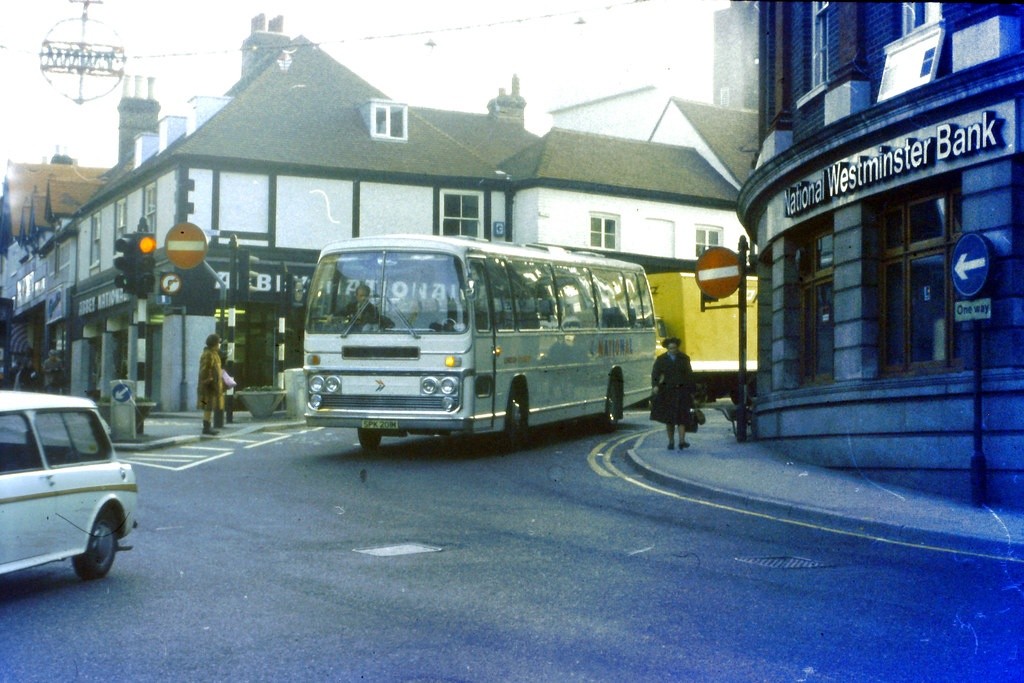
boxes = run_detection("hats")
[662,336,680,348]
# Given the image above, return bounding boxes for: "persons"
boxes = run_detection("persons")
[14,357,40,392]
[197,333,226,435]
[326,284,378,333]
[650,338,698,451]
[44,349,62,393]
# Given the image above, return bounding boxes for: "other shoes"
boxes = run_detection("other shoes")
[679,441,690,449]
[668,442,674,450]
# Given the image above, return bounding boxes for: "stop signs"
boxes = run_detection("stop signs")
[164,222,209,271]
[694,246,740,300]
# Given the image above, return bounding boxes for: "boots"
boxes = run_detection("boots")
[203,420,220,435]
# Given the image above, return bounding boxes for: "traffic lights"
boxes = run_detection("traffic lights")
[133,231,157,292]
[111,233,135,295]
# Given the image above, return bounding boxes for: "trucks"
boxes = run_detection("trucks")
[646,272,758,405]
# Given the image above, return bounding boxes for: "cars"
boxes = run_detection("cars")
[0,388,140,581]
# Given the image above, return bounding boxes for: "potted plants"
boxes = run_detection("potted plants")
[235,385,286,420]
[96,394,157,432]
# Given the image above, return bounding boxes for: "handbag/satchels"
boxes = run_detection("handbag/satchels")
[685,403,705,432]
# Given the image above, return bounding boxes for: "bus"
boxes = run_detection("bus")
[301,233,658,452]
